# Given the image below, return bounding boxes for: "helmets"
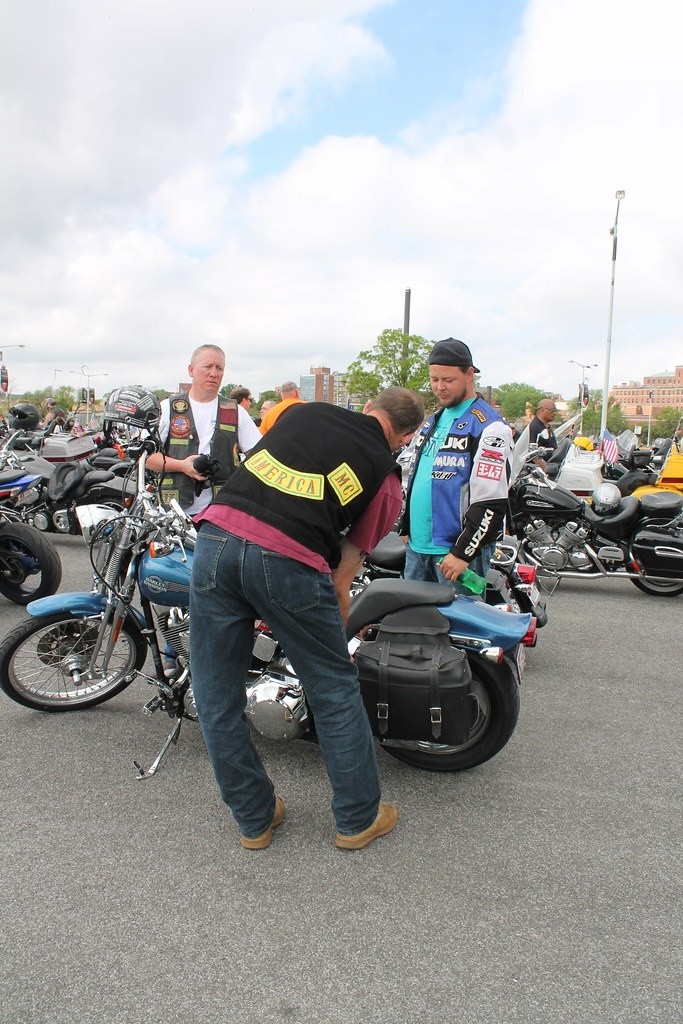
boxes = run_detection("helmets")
[103,387,161,430]
[592,483,621,516]
[8,403,40,430]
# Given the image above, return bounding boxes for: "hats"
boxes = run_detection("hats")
[428,338,480,373]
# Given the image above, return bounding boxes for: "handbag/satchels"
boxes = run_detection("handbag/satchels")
[356,606,472,745]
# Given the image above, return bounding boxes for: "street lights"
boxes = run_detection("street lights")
[569,360,599,438]
[68,370,109,426]
[597,191,627,440]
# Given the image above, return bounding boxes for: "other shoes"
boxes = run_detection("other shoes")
[335,803,397,849]
[146,662,176,685]
[240,797,284,849]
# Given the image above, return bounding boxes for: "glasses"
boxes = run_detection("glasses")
[243,397,252,404]
[539,406,557,413]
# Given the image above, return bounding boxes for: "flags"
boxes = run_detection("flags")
[603,427,618,465]
[89,412,100,431]
[76,419,84,439]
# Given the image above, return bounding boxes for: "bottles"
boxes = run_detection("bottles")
[438,556,486,594]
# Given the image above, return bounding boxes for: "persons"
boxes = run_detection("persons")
[189,388,425,850]
[135,345,264,685]
[0,413,9,439]
[260,382,308,435]
[398,341,516,603]
[529,395,559,482]
[253,386,283,428]
[643,429,681,473]
[229,387,252,411]
[39,398,66,430]
[575,431,583,441]
[592,434,598,448]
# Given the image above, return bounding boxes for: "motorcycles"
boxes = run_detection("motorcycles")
[0,426,545,774]
[0,404,683,605]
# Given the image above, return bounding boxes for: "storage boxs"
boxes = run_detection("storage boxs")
[40,433,97,468]
